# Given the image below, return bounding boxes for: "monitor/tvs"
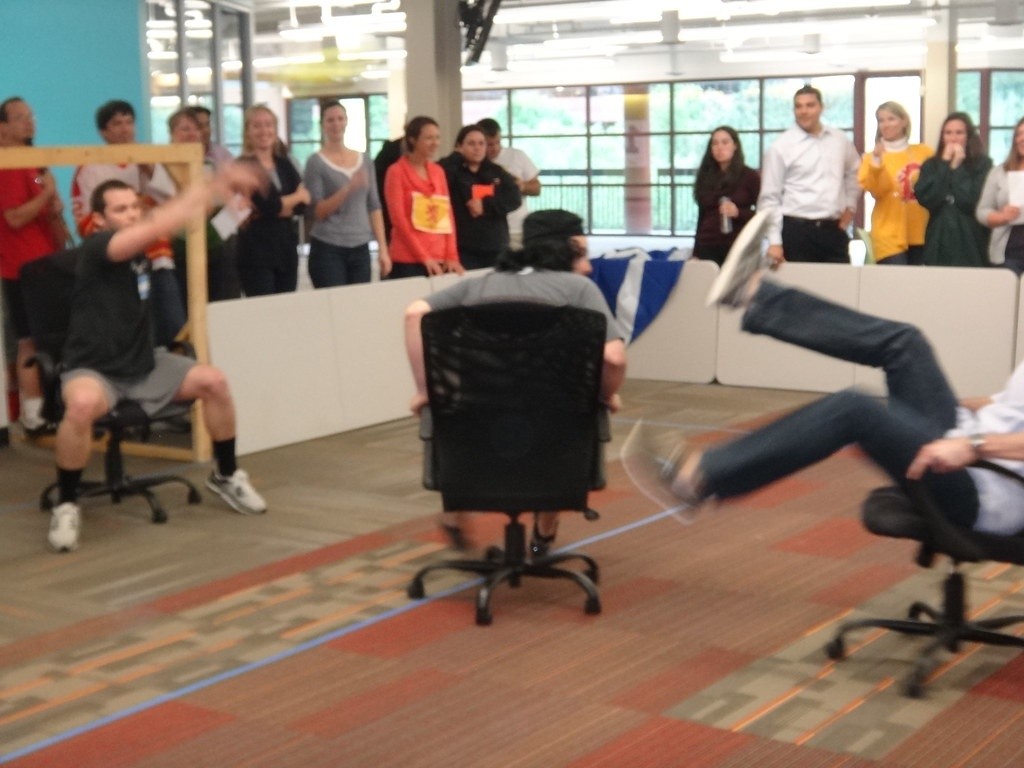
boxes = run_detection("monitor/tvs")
[465,0,502,67]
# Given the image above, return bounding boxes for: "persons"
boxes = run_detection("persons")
[913,111,996,267]
[619,208,1024,542]
[857,101,934,266]
[975,117,1024,267]
[303,102,393,290]
[0,98,65,451]
[152,107,267,305]
[46,162,268,556]
[444,126,521,271]
[403,207,627,561]
[383,116,467,280]
[689,125,762,268]
[71,101,187,354]
[759,86,862,266]
[234,105,312,295]
[475,119,541,250]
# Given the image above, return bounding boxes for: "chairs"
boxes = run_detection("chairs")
[19,248,202,525]
[826,487,1024,701]
[407,301,609,626]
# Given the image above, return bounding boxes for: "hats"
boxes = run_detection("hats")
[521,210,584,246]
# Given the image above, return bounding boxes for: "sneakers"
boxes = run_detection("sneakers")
[529,522,556,557]
[707,202,784,314]
[648,421,718,508]
[204,468,267,516]
[21,419,59,439]
[47,502,81,554]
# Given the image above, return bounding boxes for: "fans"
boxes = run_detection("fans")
[459,0,501,66]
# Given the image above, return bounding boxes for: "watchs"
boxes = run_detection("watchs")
[970,434,987,465]
